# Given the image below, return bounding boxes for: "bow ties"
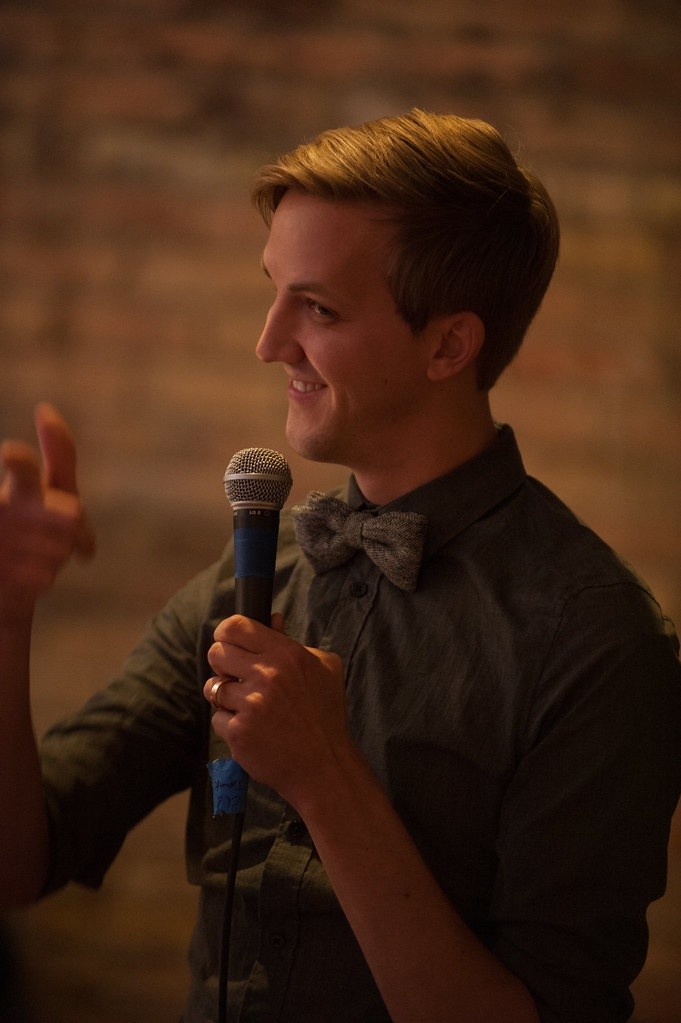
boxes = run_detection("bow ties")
[292,489,431,594]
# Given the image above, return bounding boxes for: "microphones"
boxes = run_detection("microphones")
[223,449,292,630]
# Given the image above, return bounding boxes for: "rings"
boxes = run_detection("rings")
[210,678,243,712]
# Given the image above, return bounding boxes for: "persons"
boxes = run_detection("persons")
[0,109,681,1022]
